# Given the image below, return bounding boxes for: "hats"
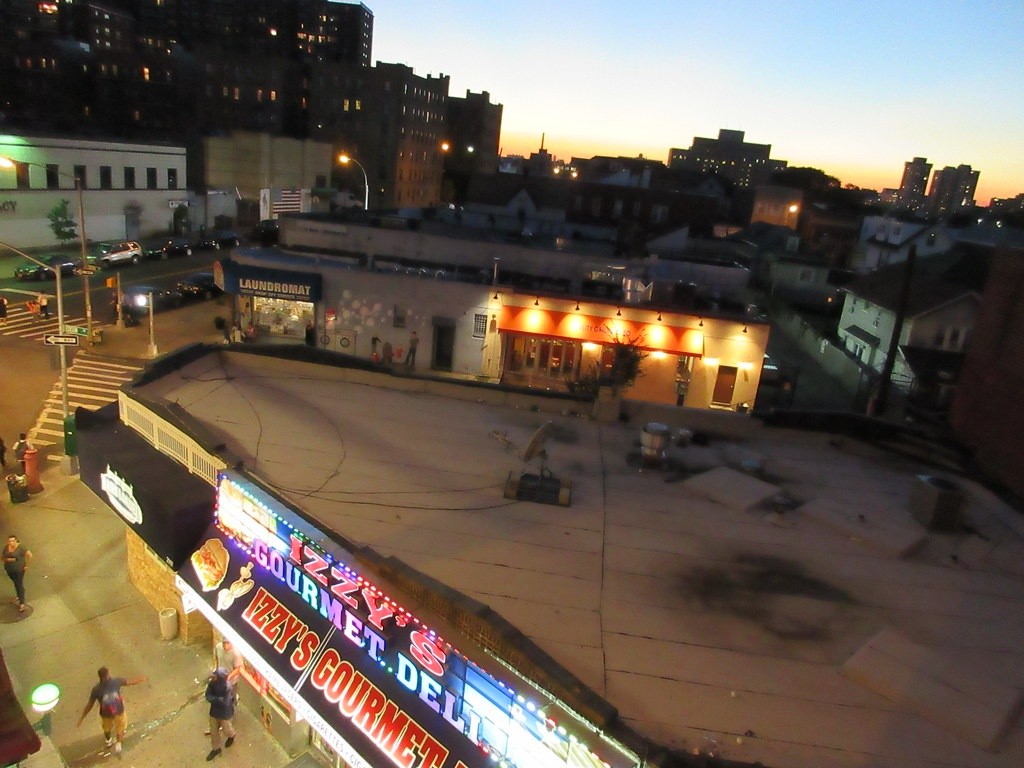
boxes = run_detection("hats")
[98,667,109,676]
[211,667,229,680]
[222,636,229,643]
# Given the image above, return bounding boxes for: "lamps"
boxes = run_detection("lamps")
[31,683,60,736]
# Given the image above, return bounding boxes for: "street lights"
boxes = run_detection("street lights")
[339,155,369,211]
[134,291,160,360]
[0,157,94,345]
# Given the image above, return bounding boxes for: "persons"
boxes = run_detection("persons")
[1,535,33,612]
[0,437,8,466]
[12,433,34,475]
[109,290,134,327]
[306,324,314,346]
[205,636,243,734]
[224,322,256,344]
[205,667,236,761]
[0,296,9,325]
[676,372,690,406]
[37,290,48,318]
[76,667,148,753]
[405,332,419,366]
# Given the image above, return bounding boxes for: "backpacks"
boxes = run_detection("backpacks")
[15,440,28,460]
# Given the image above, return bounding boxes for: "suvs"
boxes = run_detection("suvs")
[93,241,143,270]
[254,219,279,244]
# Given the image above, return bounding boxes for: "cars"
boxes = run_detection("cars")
[13,254,84,282]
[141,236,195,261]
[124,286,183,315]
[176,273,225,300]
[198,229,240,251]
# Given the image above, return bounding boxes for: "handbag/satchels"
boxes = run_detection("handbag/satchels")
[3,299,8,305]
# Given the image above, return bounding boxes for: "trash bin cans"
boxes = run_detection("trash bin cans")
[123,311,137,328]
[62,414,77,458]
[159,608,178,640]
[5,473,29,502]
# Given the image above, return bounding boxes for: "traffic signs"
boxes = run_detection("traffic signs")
[74,268,93,276]
[44,334,79,347]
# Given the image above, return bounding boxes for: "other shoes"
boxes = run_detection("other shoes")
[115,742,122,753]
[205,728,212,733]
[219,726,222,730]
[10,597,20,603]
[19,603,25,611]
[206,747,222,761]
[225,733,236,747]
[105,738,113,747]
